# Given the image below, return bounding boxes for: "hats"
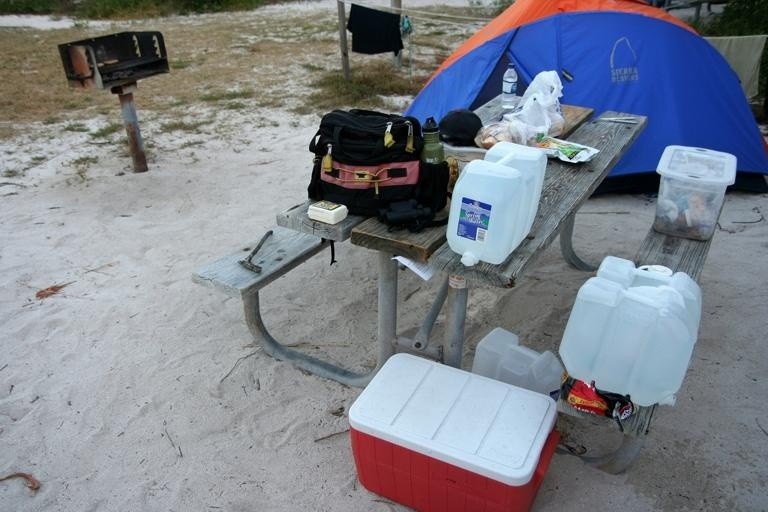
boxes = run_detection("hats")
[439,110,482,146]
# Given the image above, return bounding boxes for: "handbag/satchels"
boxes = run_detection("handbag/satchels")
[308,109,421,216]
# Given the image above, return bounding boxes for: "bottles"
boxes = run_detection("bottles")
[502,63,519,111]
[419,117,444,164]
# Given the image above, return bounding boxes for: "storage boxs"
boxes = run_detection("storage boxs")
[349,353,561,512]
[653,145,737,241]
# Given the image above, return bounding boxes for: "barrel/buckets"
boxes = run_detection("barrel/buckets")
[471,326,565,396]
[597,254,703,332]
[445,142,547,267]
[558,276,698,407]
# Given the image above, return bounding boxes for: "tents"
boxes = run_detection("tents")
[405,0,768,195]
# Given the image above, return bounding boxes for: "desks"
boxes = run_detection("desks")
[277,92,647,389]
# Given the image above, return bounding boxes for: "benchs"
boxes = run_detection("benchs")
[556,197,726,475]
[194,225,334,376]
